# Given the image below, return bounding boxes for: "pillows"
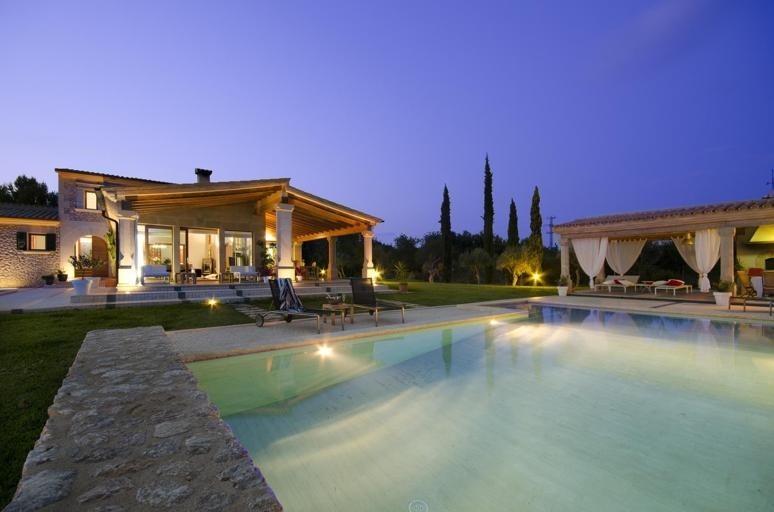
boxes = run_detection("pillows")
[664,278,686,286]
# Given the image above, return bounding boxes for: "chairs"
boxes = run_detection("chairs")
[594,275,640,293]
[737,268,774,297]
[254,276,406,333]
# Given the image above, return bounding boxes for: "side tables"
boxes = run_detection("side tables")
[175,272,196,285]
[218,272,235,284]
[641,280,654,294]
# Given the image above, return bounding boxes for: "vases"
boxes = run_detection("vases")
[263,276,268,283]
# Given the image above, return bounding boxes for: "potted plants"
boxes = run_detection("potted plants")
[712,279,735,307]
[57,254,107,296]
[556,275,571,296]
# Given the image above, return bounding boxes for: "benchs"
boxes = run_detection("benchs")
[229,266,260,283]
[654,284,693,297]
[141,264,170,285]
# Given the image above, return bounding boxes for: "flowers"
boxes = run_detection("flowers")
[261,267,273,276]
